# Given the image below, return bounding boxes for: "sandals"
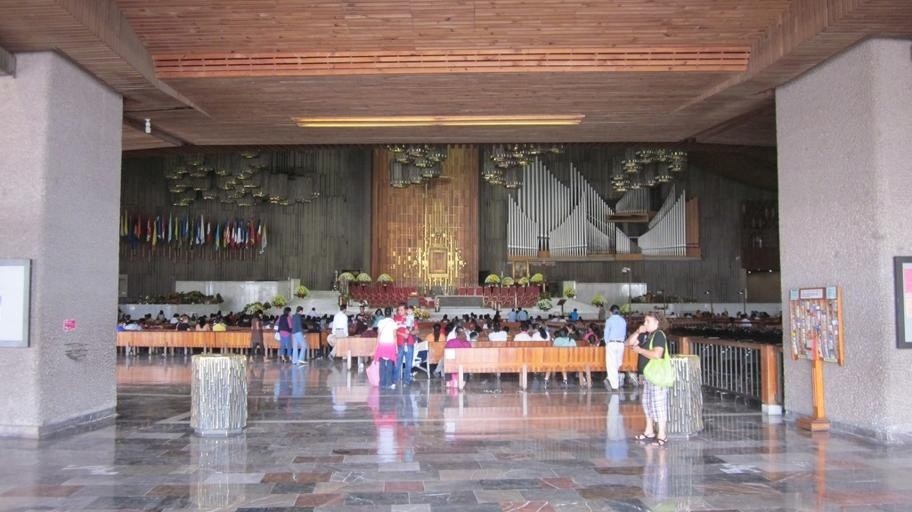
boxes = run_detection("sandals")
[635,432,668,447]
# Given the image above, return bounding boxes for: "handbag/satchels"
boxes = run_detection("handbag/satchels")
[274,331,280,341]
[366,360,380,387]
[327,333,336,347]
[643,357,675,387]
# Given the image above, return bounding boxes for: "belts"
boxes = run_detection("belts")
[609,340,624,343]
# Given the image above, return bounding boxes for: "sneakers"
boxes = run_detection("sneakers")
[467,374,501,384]
[404,347,408,352]
[328,353,334,361]
[282,356,308,364]
[531,372,587,386]
[446,380,466,388]
[381,382,407,390]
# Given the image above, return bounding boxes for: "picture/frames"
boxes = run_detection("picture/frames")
[893,255,912,349]
[0,258,33,349]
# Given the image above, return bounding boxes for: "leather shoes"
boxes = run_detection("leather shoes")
[603,379,617,392]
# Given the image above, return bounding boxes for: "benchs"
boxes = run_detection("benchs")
[116,327,327,365]
[336,327,636,392]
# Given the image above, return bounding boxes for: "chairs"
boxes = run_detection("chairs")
[458,286,540,310]
[349,285,435,308]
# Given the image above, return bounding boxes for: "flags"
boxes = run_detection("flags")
[119,205,268,254]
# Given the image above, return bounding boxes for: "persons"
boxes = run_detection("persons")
[606,388,629,465]
[625,311,673,448]
[640,443,679,512]
[364,390,466,470]
[117,294,782,389]
